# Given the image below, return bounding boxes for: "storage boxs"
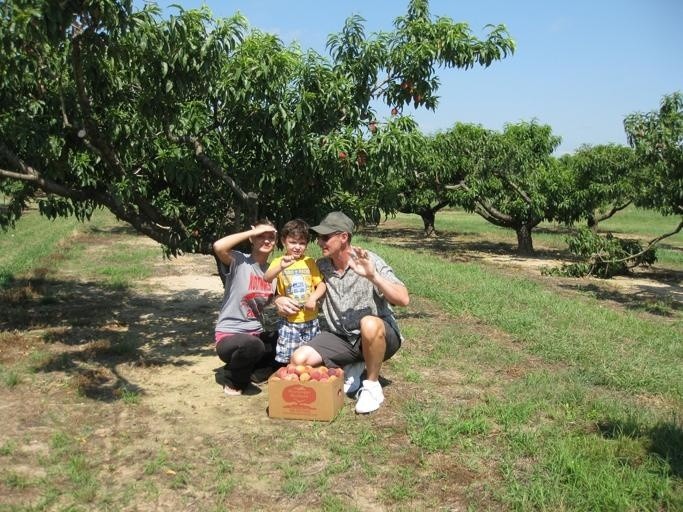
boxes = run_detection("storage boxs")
[266,366,347,422]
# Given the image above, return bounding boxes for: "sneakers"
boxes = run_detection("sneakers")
[342,361,364,394]
[355,379,383,414]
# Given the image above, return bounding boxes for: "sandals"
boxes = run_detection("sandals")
[219,371,252,396]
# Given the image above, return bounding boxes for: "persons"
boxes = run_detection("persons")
[208,218,278,396]
[262,218,327,365]
[271,207,410,415]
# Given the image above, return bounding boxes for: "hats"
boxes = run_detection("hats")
[309,211,354,238]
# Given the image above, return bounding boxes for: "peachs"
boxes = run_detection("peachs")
[400,82,408,89]
[339,152,346,159]
[270,363,342,384]
[415,95,421,102]
[370,124,377,133]
[392,107,397,115]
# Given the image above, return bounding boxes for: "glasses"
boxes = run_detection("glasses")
[315,232,343,241]
